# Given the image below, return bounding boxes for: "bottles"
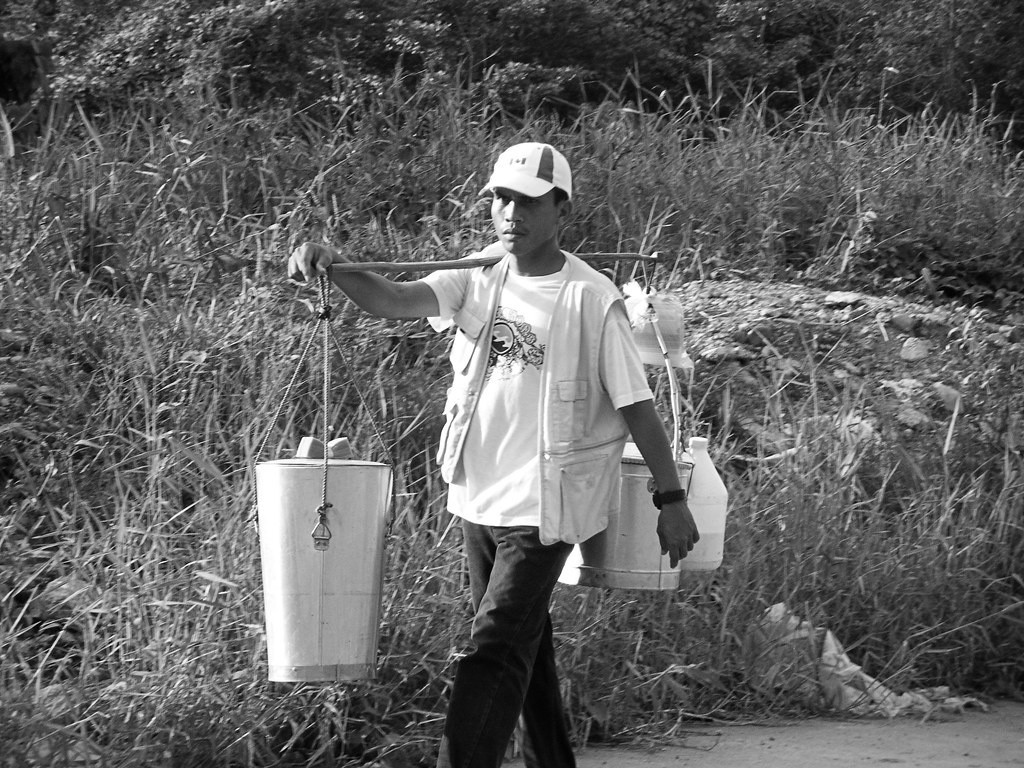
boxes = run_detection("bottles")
[681,437,727,571]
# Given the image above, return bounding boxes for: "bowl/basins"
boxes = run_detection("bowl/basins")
[632,304,683,367]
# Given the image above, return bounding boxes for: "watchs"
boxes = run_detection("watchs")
[653,490,687,510]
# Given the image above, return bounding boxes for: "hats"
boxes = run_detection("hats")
[478,142,572,202]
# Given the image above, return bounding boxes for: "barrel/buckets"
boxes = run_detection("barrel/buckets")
[577,442,694,589]
[255,459,390,682]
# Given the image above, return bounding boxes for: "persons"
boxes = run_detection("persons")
[286,141,701,768]
